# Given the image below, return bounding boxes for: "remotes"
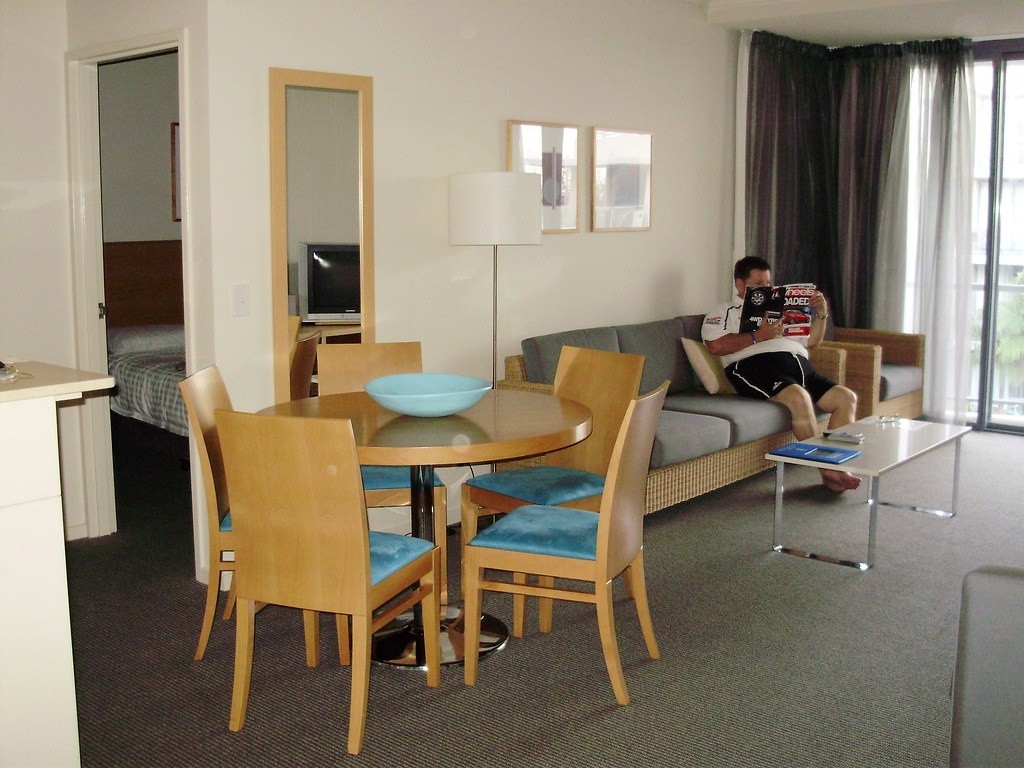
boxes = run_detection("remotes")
[823,431,864,444]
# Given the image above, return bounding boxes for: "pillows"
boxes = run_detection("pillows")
[107,323,186,355]
[680,338,738,395]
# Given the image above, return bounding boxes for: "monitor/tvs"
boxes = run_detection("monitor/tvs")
[299,241,360,325]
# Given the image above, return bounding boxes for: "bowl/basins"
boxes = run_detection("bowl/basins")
[364,372,493,417]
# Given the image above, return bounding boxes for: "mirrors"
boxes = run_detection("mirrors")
[268,66,376,404]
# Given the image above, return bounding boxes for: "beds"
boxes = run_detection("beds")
[102,240,189,437]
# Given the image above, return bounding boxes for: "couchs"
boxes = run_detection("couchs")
[819,303,926,422]
[494,312,849,518]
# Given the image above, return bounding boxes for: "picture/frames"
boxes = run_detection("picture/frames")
[590,126,654,232]
[171,122,182,222]
[506,119,580,234]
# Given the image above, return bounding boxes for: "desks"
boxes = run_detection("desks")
[0,361,117,768]
[256,389,594,671]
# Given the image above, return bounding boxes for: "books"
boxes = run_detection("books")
[739,282,817,338]
[769,443,860,465]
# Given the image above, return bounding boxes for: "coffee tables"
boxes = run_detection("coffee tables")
[764,415,973,571]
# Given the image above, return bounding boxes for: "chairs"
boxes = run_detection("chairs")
[178,364,353,667]
[290,329,321,400]
[214,407,443,756]
[462,345,647,640]
[317,340,449,607]
[289,314,301,360]
[461,378,671,707]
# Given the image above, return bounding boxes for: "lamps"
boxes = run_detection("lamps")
[446,169,541,386]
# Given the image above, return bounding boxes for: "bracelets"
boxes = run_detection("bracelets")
[815,312,828,320]
[750,330,756,345]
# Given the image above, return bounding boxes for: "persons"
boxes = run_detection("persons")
[701,256,862,492]
